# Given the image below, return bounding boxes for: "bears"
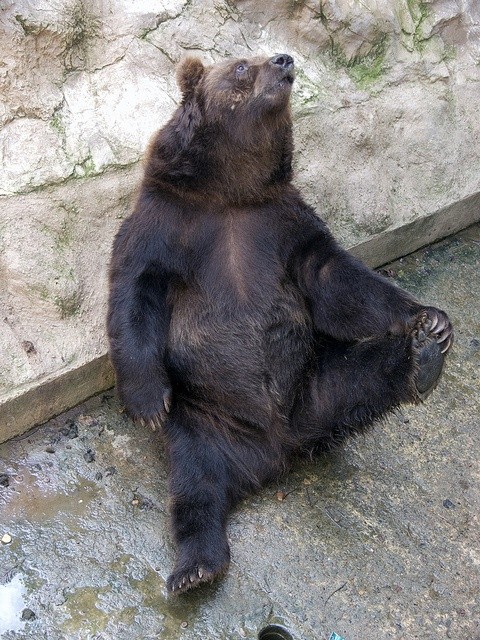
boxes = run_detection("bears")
[107,54,454,596]
